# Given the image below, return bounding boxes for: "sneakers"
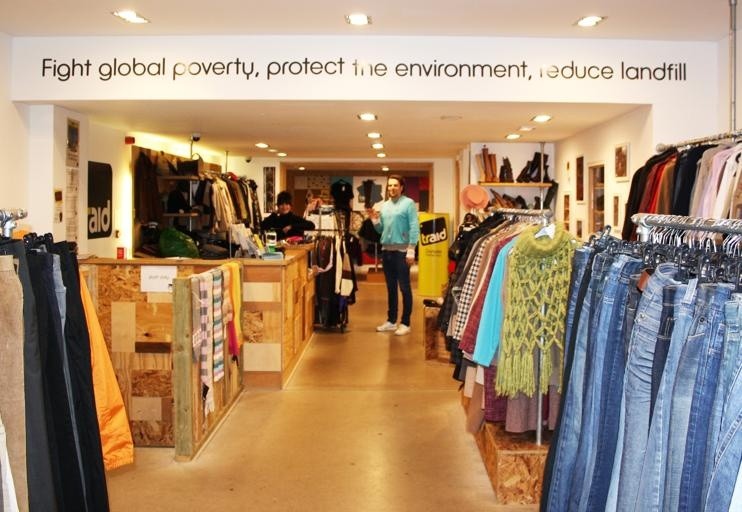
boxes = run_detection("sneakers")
[375,320,411,336]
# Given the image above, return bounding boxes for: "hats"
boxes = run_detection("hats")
[459,185,492,212]
[277,190,292,206]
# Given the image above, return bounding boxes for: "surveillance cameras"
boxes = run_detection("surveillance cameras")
[191,133,200,141]
[246,156,252,163]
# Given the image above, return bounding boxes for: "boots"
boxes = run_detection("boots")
[531,154,548,183]
[482,148,493,182]
[523,152,542,182]
[543,165,551,182]
[503,158,515,182]
[516,160,530,182]
[488,154,499,182]
[499,165,510,182]
[475,153,486,183]
[473,181,559,209]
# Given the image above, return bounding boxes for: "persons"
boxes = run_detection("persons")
[368,175,420,335]
[261,191,315,242]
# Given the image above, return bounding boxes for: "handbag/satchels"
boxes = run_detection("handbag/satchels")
[160,226,201,258]
[339,277,358,304]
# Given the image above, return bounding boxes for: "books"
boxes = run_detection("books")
[262,252,283,260]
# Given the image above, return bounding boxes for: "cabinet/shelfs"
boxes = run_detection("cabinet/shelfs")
[158,173,203,218]
[480,181,552,214]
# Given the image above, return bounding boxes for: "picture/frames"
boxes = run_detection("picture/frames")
[612,141,633,184]
